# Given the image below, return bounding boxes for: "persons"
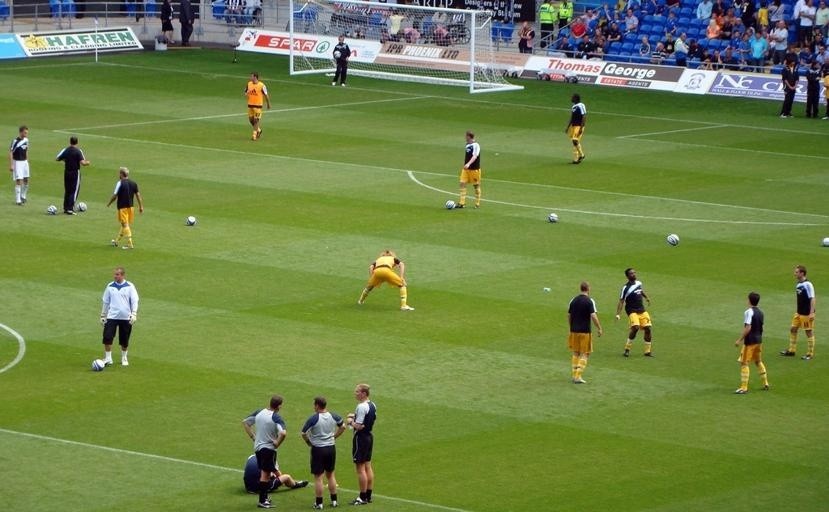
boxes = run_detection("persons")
[160,0,263,47]
[780,264,817,361]
[455,130,481,209]
[243,394,288,509]
[564,93,587,163]
[107,166,142,251]
[56,136,90,214]
[242,454,308,494]
[9,126,31,206]
[244,73,273,141]
[355,246,412,312]
[99,268,140,367]
[734,293,771,394]
[346,382,377,505]
[302,396,346,509]
[615,268,655,357]
[332,1,829,117]
[566,279,603,386]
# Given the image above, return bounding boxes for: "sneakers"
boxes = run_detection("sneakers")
[103,360,112,365]
[252,129,262,141]
[258,480,373,510]
[122,356,129,366]
[400,306,414,310]
[572,348,814,395]
[16,194,134,250]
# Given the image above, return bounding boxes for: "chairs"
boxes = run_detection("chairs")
[545,0,829,78]
[285,2,518,41]
[1,0,262,24]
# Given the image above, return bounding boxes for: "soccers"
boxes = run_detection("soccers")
[92,359,104,371]
[186,216,195,225]
[446,200,455,209]
[77,202,87,212]
[667,234,679,246]
[48,206,57,216]
[334,50,341,58]
[549,213,558,222]
[822,238,829,246]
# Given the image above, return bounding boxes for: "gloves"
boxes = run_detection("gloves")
[128,314,136,326]
[100,316,106,326]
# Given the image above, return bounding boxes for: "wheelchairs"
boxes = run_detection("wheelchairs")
[447,20,471,45]
